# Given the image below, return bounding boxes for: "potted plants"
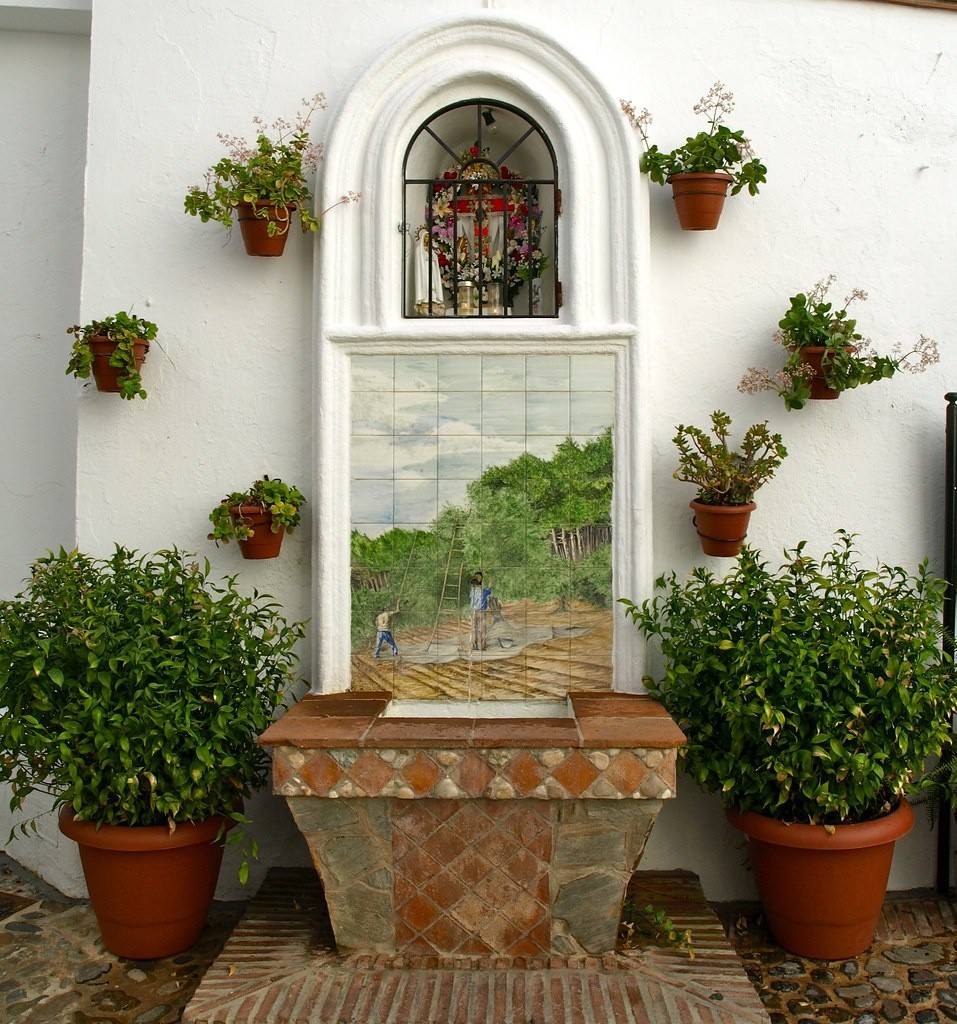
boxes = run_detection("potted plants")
[65,311,158,401]
[619,528,957,962]
[206,480,308,560]
[673,409,789,557]
[0,543,310,961]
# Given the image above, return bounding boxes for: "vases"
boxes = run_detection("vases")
[667,173,732,230]
[788,346,855,400]
[235,200,297,257]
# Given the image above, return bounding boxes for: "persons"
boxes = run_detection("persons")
[414,228,443,304]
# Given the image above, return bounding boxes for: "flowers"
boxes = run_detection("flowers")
[426,142,551,309]
[736,273,941,412]
[619,79,768,197]
[183,92,365,238]
